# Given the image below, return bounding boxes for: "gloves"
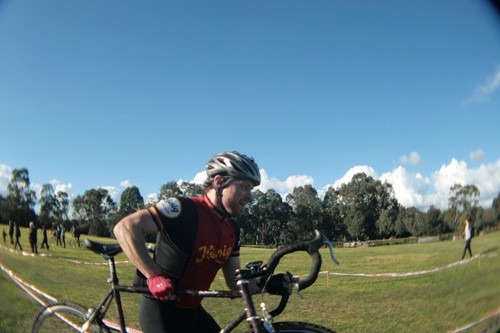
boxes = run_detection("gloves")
[147,274,173,301]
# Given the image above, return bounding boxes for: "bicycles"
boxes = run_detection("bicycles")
[33,228,341,333]
[69,239,85,250]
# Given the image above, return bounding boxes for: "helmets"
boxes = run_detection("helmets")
[206,150,261,185]
